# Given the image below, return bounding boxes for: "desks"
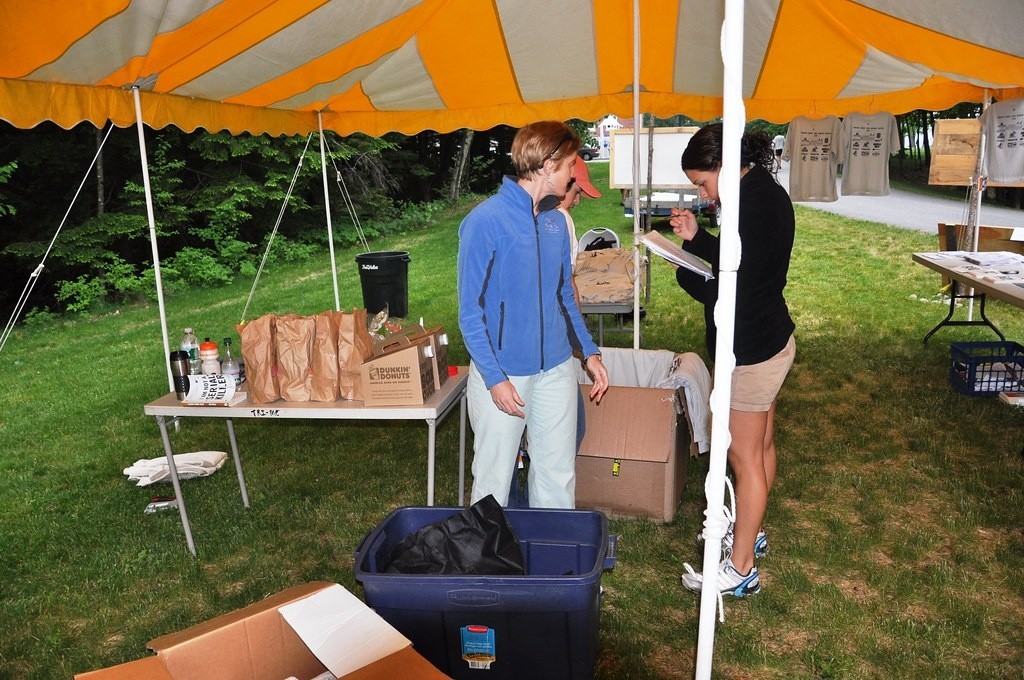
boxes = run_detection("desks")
[143,364,470,559]
[911,251,1024,345]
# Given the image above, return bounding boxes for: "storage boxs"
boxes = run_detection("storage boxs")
[947,341,1024,398]
[936,221,1024,297]
[73,580,453,680]
[352,507,617,680]
[575,344,714,526]
[360,325,449,407]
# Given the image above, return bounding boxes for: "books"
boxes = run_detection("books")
[181,373,247,408]
[632,229,716,282]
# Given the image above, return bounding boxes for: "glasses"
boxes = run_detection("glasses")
[539,130,573,169]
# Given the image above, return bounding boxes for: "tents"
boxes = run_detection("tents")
[0,0,1024,680]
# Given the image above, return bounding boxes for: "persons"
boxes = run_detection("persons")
[457,120,610,597]
[668,122,798,598]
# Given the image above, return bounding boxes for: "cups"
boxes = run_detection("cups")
[170,351,191,401]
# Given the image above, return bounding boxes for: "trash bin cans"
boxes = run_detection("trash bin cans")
[354,250,413,319]
[351,503,617,679]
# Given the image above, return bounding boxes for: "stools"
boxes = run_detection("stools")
[573,245,634,350]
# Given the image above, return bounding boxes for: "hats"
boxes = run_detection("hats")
[570,155,602,199]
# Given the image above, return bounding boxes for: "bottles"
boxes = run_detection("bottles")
[180,327,201,375]
[200,337,221,375]
[221,338,242,391]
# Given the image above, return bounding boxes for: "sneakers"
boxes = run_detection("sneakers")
[697,523,769,558]
[682,558,761,597]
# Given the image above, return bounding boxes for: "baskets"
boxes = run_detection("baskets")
[948,341,1024,397]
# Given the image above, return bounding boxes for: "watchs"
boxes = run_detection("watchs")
[584,352,602,362]
